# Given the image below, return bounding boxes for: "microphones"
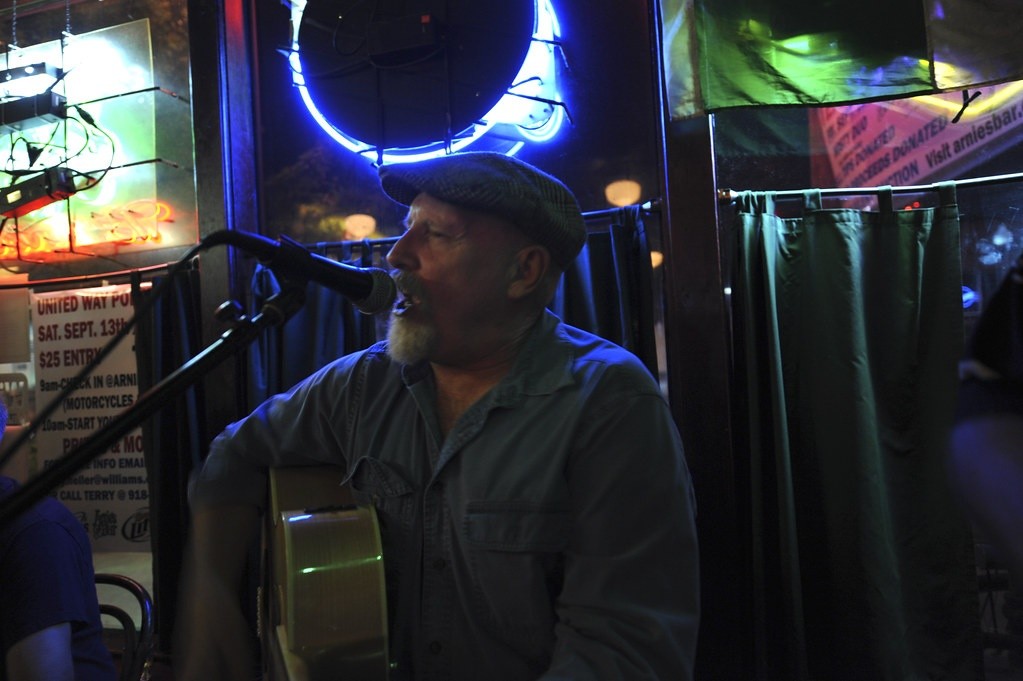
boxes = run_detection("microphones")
[232,229,397,315]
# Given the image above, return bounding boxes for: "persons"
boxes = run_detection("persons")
[0,397,117,681]
[174,151,698,681]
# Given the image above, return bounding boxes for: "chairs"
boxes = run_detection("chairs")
[90,571,156,681]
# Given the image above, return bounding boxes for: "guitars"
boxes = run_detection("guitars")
[257,460,393,681]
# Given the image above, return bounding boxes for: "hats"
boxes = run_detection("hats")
[379,152,587,268]
